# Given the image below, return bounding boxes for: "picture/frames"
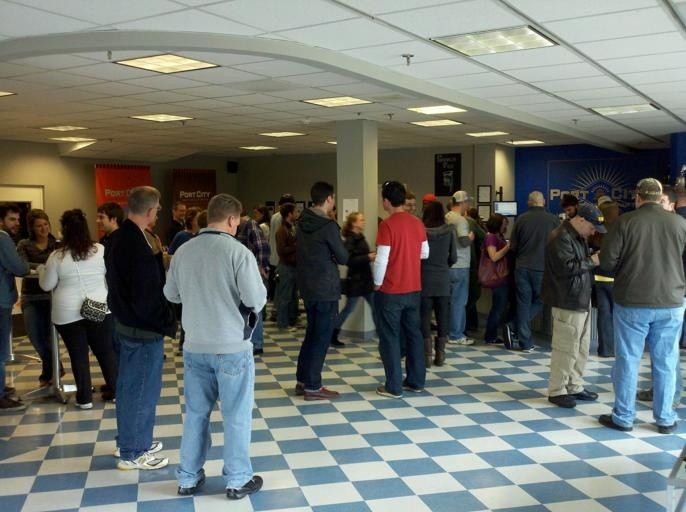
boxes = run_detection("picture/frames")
[477,205,491,223]
[0,184,45,248]
[477,185,491,203]
[295,201,306,220]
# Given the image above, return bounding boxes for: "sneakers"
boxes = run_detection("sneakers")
[450,327,535,353]
[227,476,263,498]
[2,385,166,469]
[178,476,205,494]
[402,385,425,392]
[376,384,402,398]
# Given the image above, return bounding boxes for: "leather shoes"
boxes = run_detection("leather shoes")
[549,388,677,432]
[295,383,340,401]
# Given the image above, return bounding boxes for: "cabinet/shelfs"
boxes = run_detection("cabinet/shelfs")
[434,153,461,196]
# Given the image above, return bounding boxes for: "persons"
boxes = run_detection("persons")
[269,177,686,432]
[0,177,270,502]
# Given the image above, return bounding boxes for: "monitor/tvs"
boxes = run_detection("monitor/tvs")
[493,201,518,217]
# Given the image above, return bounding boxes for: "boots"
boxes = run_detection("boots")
[425,336,447,365]
[333,330,345,347]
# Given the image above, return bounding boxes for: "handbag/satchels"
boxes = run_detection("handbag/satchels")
[478,253,510,288]
[80,298,107,323]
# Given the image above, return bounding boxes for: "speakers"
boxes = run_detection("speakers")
[227,161,238,173]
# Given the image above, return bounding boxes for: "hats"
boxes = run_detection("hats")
[578,203,608,233]
[453,190,473,202]
[630,178,662,195]
[423,193,436,203]
